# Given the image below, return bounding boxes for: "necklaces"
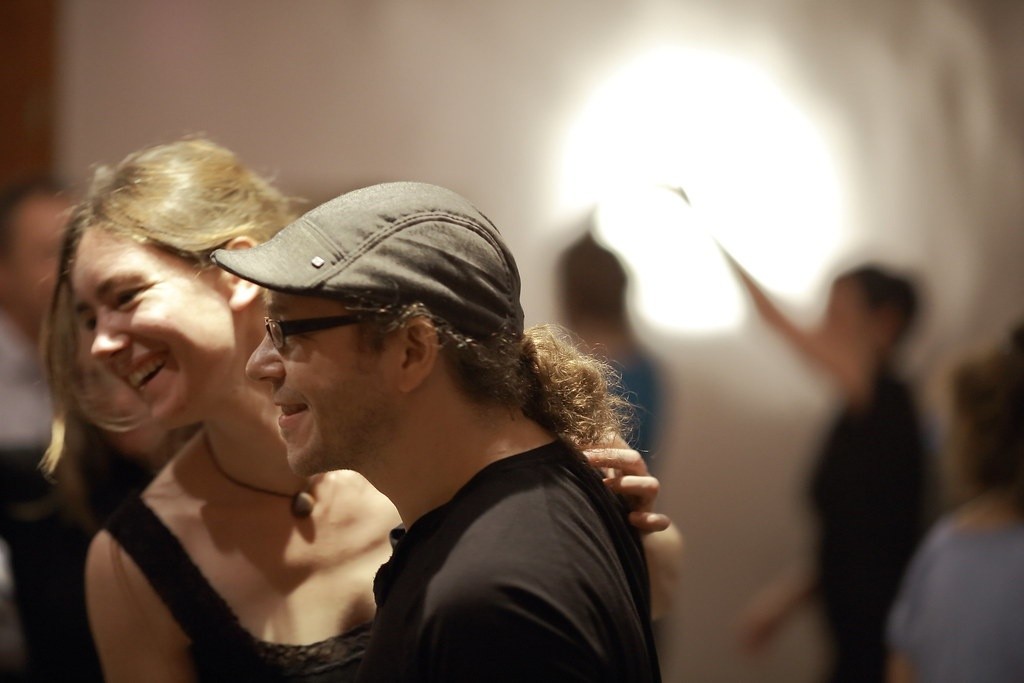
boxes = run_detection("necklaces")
[203,430,315,516]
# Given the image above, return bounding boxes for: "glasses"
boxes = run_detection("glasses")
[264,312,393,350]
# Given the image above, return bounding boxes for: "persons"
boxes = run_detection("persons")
[887,329,1024,683]
[560,235,659,470]
[0,172,106,683]
[215,180,663,683]
[674,184,941,683]
[39,138,670,683]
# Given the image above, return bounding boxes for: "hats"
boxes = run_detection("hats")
[210,182,524,344]
